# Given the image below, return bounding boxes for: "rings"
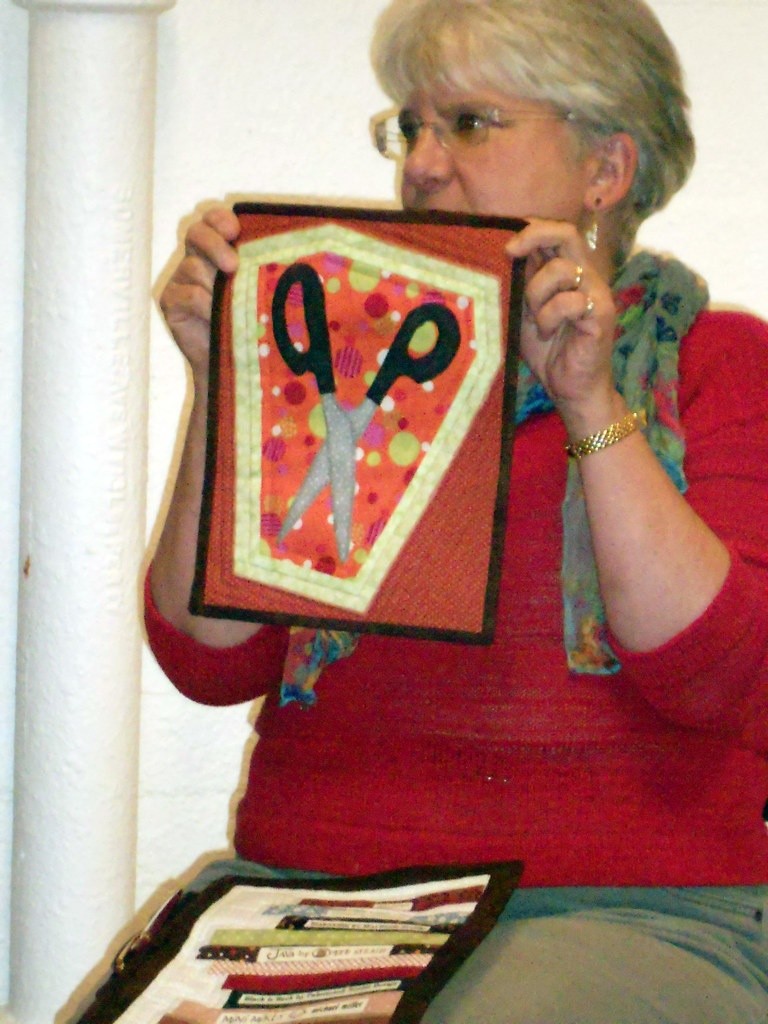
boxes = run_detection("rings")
[571,265,583,290]
[582,293,594,321]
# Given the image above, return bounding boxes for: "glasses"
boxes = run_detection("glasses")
[372,103,577,158]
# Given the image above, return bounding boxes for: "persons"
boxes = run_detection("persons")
[142,0,768,1024]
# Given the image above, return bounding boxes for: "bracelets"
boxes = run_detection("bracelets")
[564,414,637,460]
